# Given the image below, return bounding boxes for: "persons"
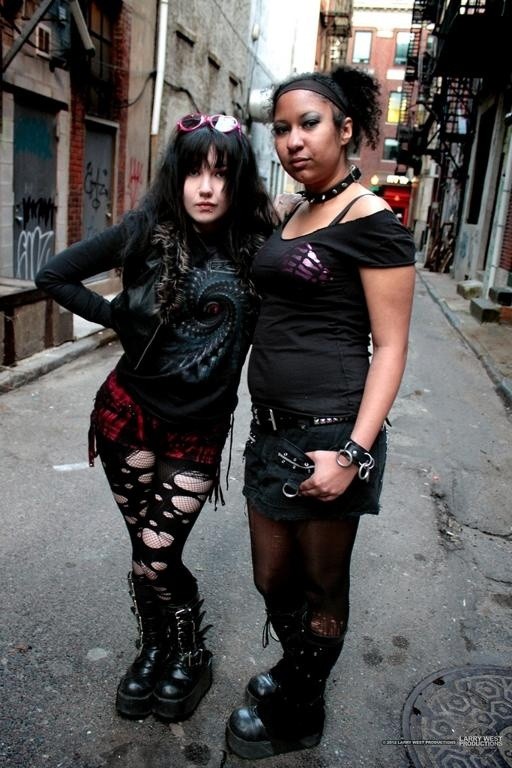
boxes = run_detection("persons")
[35,114,302,720]
[226,67,414,759]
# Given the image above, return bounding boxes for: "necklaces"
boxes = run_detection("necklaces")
[303,164,362,203]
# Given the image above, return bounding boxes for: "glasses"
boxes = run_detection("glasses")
[176,112,241,141]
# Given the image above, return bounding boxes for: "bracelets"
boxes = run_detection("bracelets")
[337,438,377,480]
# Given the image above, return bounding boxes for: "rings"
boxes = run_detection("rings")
[279,199,284,204]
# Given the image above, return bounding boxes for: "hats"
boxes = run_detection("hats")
[261,440,314,508]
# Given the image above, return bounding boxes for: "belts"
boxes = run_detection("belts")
[253,411,361,432]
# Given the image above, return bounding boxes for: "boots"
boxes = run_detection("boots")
[114,574,212,722]
[226,611,347,761]
